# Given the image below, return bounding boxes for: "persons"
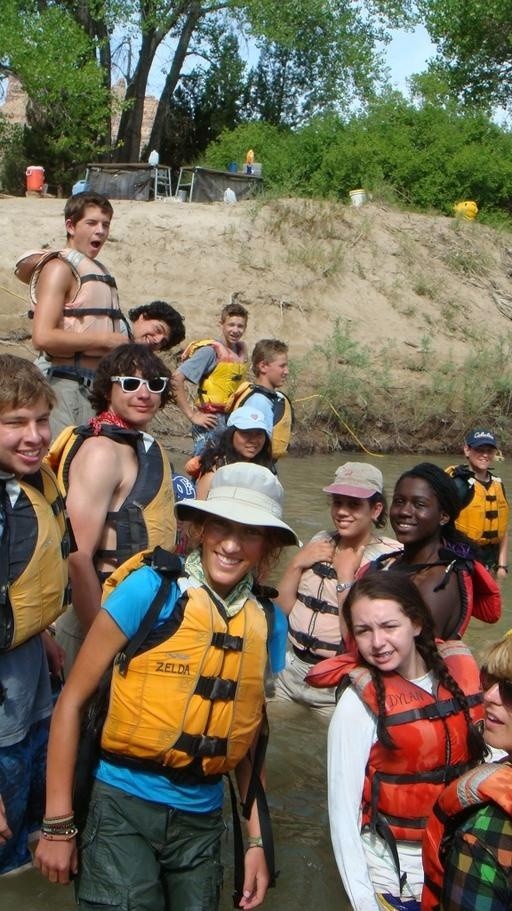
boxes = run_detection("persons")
[324,572,483,911]
[441,428,510,586]
[25,191,126,440]
[227,338,294,463]
[174,304,251,457]
[305,461,502,703]
[424,630,512,908]
[60,345,175,817]
[122,300,185,356]
[274,459,406,722]
[33,461,302,908]
[0,353,79,878]
[184,406,271,477]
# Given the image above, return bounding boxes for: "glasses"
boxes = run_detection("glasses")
[480,667,512,708]
[112,375,170,396]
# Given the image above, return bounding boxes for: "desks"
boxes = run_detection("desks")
[175,165,264,203]
[84,162,172,202]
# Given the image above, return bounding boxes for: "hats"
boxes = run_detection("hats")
[322,462,384,497]
[228,405,271,434]
[464,428,499,450]
[174,463,306,550]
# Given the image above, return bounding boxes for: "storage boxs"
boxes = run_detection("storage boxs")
[243,163,262,176]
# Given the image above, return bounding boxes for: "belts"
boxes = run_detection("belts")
[48,369,95,391]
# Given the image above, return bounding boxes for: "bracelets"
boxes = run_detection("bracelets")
[244,835,266,849]
[41,815,75,843]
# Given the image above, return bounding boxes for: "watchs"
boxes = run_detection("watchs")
[497,564,509,571]
[335,583,355,592]
